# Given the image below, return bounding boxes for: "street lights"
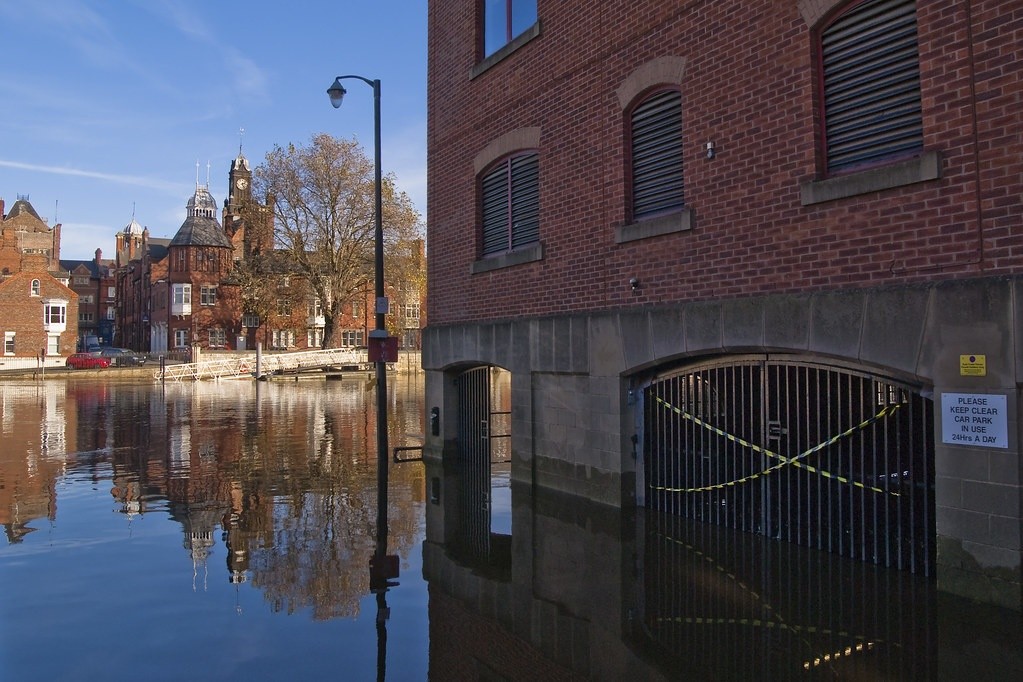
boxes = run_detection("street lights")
[324,73,389,475]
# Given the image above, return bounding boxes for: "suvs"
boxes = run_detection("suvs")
[98,348,147,367]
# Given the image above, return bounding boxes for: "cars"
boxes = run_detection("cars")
[66,352,109,369]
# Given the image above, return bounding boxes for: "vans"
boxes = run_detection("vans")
[80,335,100,353]
